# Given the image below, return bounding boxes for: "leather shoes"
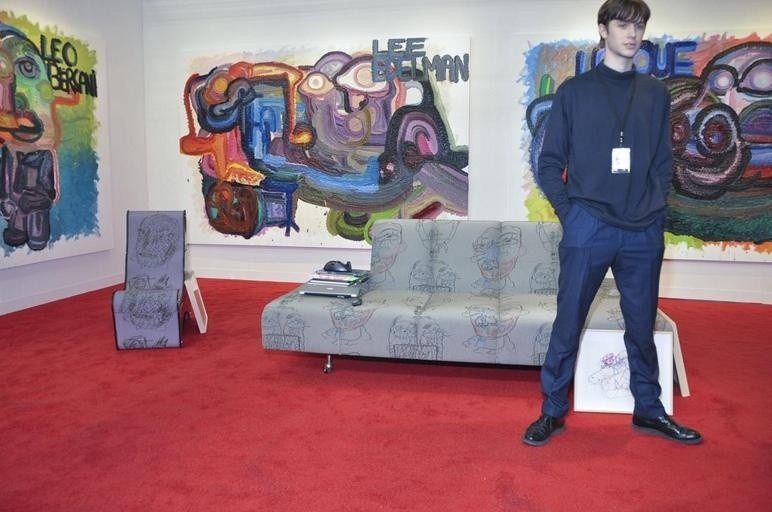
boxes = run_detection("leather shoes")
[523,413,564,445]
[632,413,703,444]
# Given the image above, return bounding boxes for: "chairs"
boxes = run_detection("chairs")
[111,209,187,350]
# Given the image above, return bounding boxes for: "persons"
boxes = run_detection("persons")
[522,0,702,448]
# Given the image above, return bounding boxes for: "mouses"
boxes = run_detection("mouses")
[324,260,352,273]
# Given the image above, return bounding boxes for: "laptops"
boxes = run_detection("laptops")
[298,283,362,298]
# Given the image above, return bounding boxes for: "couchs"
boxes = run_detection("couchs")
[260,219,667,375]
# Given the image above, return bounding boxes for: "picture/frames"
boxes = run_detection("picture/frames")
[185,275,209,333]
[571,328,675,417]
